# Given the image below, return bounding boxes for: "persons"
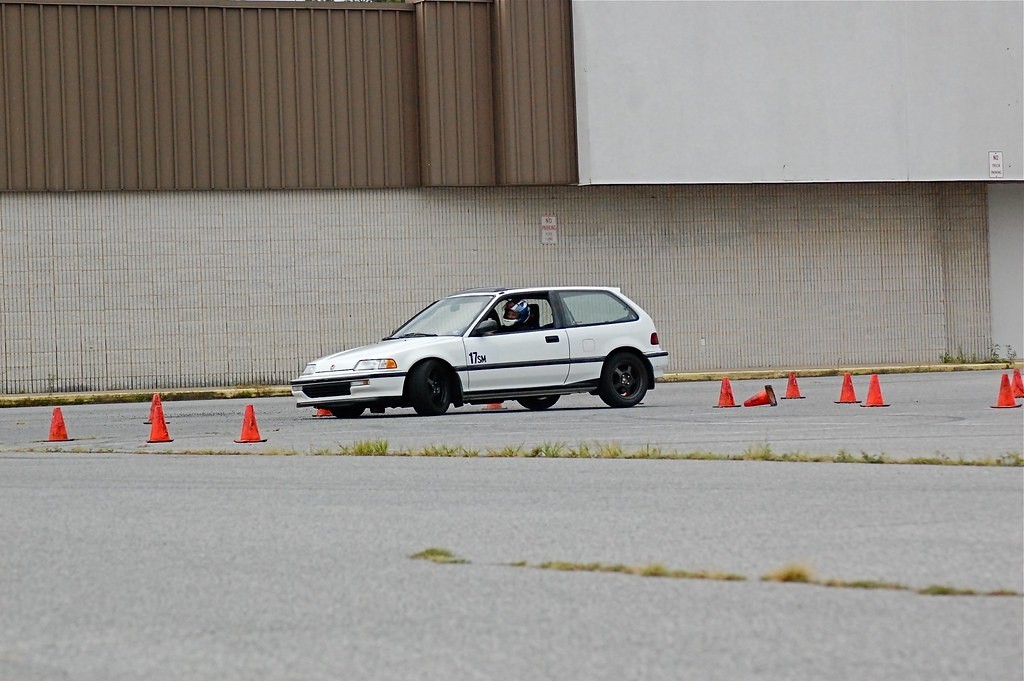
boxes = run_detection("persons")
[492,298,531,333]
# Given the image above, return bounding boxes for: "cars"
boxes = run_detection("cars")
[285,283,670,420]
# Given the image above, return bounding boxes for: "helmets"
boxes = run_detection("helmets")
[502,298,531,327]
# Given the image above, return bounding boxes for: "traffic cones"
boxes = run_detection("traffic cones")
[143,394,171,424]
[146,403,174,443]
[1011,368,1024,398]
[41,407,75,442]
[988,372,1022,408]
[743,385,778,407]
[834,373,862,403]
[780,370,806,399]
[481,403,508,411]
[311,409,333,417]
[232,404,268,443]
[860,373,891,407]
[711,377,742,408]
